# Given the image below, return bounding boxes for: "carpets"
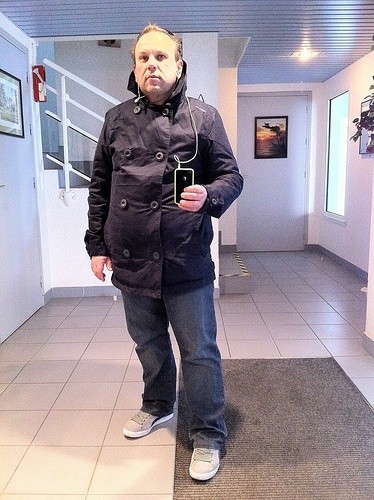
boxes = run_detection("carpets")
[173,357,374,500]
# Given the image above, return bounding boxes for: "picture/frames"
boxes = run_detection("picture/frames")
[254,116,288,159]
[0,69,25,138]
[359,99,372,154]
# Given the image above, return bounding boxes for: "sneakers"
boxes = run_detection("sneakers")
[123,410,174,438]
[189,448,219,480]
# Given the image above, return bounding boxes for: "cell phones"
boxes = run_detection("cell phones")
[174,168,194,204]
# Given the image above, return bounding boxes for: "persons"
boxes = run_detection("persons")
[83,24,244,483]
